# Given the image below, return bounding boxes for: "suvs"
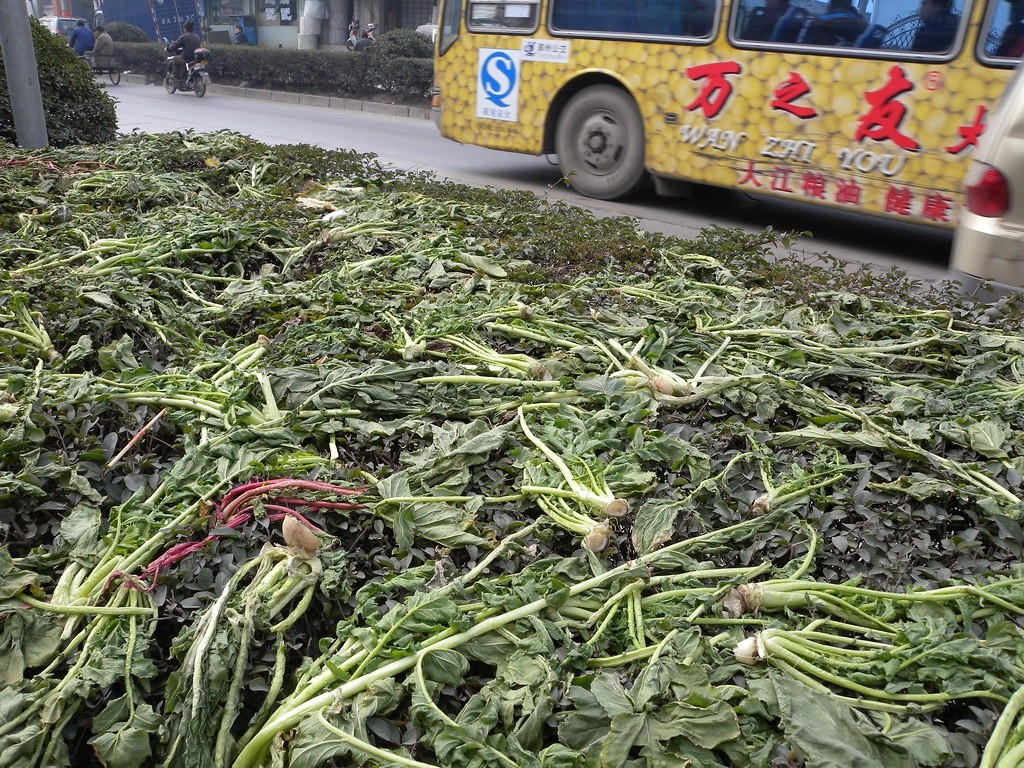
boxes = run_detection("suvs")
[37,15,94,43]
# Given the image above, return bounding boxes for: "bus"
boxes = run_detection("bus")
[432,0,1024,242]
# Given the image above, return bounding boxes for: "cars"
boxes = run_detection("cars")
[946,53,1024,319]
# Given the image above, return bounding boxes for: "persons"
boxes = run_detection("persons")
[818,0,864,42]
[163,21,201,79]
[909,1,961,54]
[233,24,248,47]
[84,26,115,56]
[69,17,95,58]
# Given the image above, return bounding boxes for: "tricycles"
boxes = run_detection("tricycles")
[69,45,125,86]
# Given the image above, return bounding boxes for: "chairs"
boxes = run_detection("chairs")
[744,6,888,50]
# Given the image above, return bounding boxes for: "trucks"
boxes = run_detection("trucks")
[51,0,206,49]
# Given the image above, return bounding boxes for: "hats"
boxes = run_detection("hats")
[93,24,104,31]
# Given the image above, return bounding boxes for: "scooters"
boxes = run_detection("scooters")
[161,36,212,97]
[345,14,379,52]
